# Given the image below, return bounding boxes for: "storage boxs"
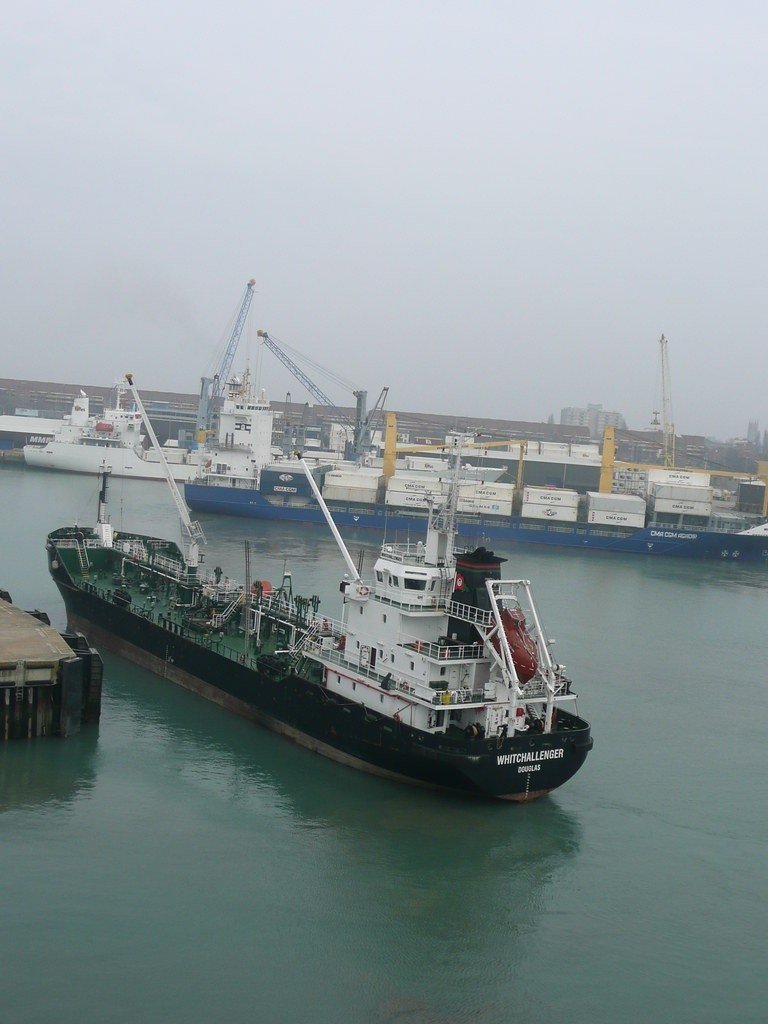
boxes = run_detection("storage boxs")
[143,446,210,465]
[322,436,714,528]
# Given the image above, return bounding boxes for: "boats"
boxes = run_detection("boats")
[42,438,595,803]
[21,381,213,484]
[183,410,768,561]
[492,606,540,683]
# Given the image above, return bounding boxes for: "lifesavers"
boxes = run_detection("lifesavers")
[360,587,369,596]
[402,682,409,691]
